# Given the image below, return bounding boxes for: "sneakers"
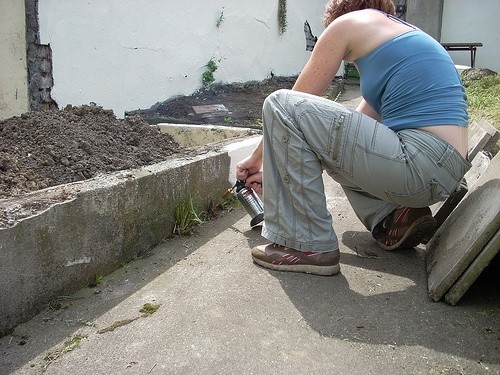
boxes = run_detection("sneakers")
[376,206,437,251]
[251,242,340,276]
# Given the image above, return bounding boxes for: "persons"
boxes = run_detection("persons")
[234,0,473,277]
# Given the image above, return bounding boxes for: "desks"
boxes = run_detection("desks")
[439,42,483,69]
